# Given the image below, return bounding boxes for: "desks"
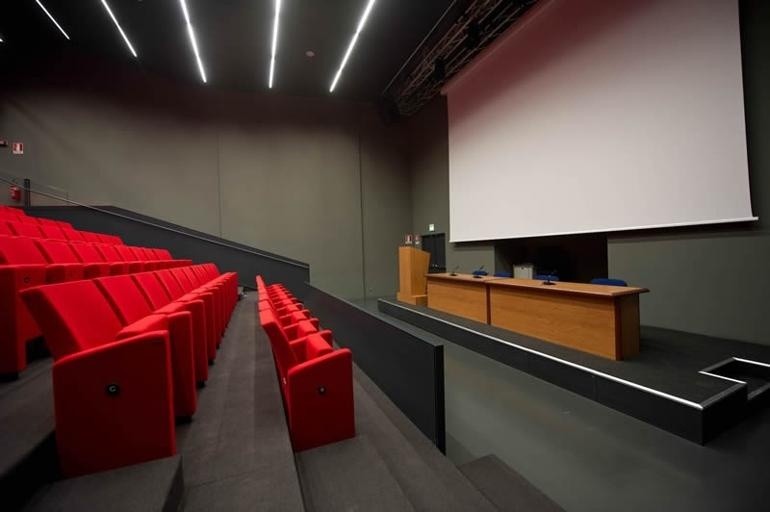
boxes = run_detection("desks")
[423,271,512,326]
[484,278,651,362]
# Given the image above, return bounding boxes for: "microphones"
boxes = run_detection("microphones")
[449,265,459,276]
[543,269,557,285]
[473,264,484,278]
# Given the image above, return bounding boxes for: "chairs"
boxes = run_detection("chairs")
[493,271,513,277]
[591,278,625,286]
[471,270,488,275]
[0,203,194,376]
[255,272,356,453]
[533,274,559,281]
